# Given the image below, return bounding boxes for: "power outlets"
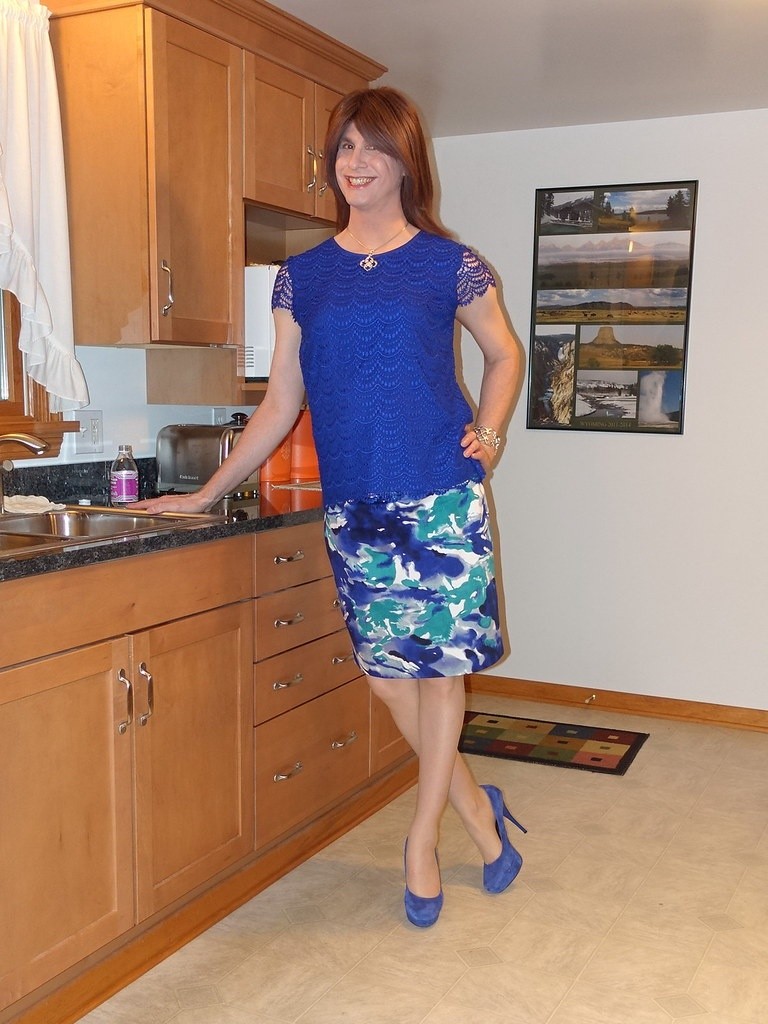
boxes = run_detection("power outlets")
[73,410,104,453]
[212,408,227,426]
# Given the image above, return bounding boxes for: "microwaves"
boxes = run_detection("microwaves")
[244,265,283,380]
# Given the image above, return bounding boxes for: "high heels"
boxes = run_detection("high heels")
[404,836,443,927]
[479,785,527,893]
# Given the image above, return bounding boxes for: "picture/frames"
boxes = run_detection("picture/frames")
[526,181,698,435]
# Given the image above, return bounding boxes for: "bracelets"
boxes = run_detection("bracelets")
[471,423,501,450]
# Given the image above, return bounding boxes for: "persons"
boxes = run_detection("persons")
[127,87,529,927]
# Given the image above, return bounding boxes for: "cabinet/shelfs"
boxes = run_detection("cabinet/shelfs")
[255,519,371,849]
[240,47,346,224]
[0,599,255,1001]
[45,6,244,347]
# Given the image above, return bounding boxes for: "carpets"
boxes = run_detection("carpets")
[458,711,649,777]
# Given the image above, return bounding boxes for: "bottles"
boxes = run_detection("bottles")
[110,445,138,508]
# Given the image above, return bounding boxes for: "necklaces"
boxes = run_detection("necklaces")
[346,221,409,271]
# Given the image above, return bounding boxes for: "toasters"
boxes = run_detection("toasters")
[156,424,260,498]
